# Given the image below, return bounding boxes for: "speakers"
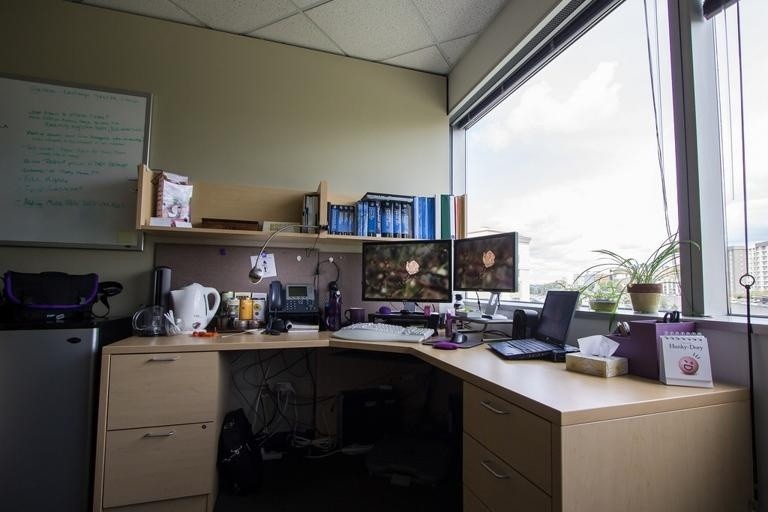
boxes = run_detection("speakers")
[426,314,439,337]
[512,309,539,339]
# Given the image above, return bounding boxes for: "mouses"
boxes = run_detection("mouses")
[451,332,465,344]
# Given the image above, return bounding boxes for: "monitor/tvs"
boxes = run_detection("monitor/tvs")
[362,240,452,314]
[454,231,519,319]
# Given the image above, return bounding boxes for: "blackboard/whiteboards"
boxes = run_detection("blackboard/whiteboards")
[0,74,154,252]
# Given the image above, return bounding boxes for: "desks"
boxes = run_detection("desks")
[93,322,757,511]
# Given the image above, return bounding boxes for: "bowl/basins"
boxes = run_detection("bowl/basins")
[164,319,186,336]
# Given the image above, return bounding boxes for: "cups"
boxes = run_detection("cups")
[248,321,263,329]
[240,299,252,320]
[227,297,240,319]
[345,307,367,324]
[221,292,234,315]
[232,320,249,330]
[252,298,265,320]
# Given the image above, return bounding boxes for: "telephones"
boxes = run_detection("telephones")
[266,281,319,313]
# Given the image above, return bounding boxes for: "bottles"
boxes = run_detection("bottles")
[328,287,341,330]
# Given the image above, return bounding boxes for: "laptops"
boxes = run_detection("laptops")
[487,291,580,360]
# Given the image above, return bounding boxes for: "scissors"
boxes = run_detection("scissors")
[664,311,680,323]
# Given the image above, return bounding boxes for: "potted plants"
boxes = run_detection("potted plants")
[586,269,622,312]
[574,229,703,312]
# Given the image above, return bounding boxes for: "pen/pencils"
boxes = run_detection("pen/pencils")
[614,321,631,337]
[218,331,244,333]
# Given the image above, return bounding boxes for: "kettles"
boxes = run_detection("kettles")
[169,282,221,333]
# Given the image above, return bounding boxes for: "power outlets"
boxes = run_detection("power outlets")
[249,253,277,279]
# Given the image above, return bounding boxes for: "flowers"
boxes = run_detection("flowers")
[402,260,422,279]
[477,248,497,279]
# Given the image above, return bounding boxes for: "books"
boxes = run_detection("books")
[287,328,319,334]
[300,193,465,242]
[291,320,320,329]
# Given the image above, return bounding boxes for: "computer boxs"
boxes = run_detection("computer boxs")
[337,388,403,470]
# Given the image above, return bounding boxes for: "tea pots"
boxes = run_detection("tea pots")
[132,304,167,336]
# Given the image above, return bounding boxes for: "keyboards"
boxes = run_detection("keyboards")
[331,322,435,343]
